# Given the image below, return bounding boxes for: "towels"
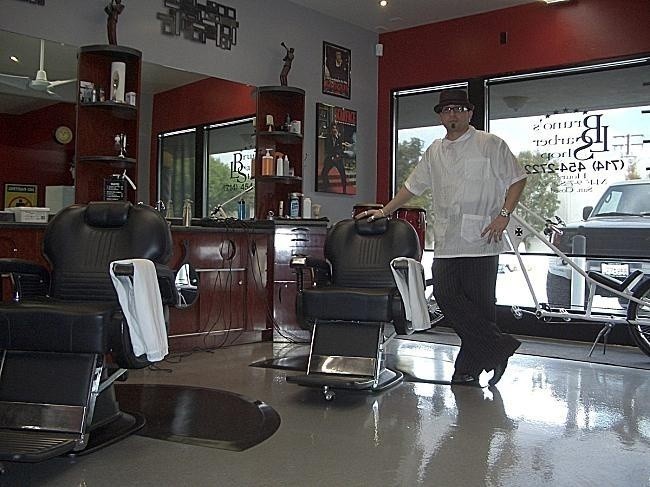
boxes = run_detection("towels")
[390,256,432,332]
[109,259,169,362]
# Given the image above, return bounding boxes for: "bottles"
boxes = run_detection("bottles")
[238,199,246,220]
[303,198,312,219]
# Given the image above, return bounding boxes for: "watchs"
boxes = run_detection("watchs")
[501,207,510,217]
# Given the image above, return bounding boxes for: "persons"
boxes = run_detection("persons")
[322,123,348,193]
[279,42,295,85]
[332,49,345,77]
[354,88,530,387]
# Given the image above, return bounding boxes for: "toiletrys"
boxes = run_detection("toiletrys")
[283,154,289,176]
[276,154,284,176]
[279,200,284,215]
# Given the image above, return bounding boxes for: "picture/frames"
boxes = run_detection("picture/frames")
[322,40,351,99]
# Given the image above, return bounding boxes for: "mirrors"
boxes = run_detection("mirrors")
[0,27,78,220]
[138,59,258,221]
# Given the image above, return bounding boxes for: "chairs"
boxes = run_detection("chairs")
[284,215,421,401]
[0,201,178,463]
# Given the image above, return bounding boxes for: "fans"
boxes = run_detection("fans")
[0,38,78,97]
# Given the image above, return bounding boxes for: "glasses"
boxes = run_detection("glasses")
[440,106,472,113]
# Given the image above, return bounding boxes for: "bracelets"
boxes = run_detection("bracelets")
[379,208,384,217]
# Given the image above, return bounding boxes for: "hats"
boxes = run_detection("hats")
[434,89,474,114]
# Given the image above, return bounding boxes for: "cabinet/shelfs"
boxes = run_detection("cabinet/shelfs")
[269,223,329,342]
[74,45,143,206]
[255,86,306,222]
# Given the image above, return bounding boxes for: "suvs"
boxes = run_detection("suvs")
[546,178,650,307]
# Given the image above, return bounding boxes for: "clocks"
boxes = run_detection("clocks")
[52,124,73,145]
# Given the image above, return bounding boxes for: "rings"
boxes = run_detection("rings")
[371,215,375,220]
[364,211,367,215]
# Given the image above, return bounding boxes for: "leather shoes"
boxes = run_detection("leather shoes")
[451,373,476,382]
[488,342,521,387]
